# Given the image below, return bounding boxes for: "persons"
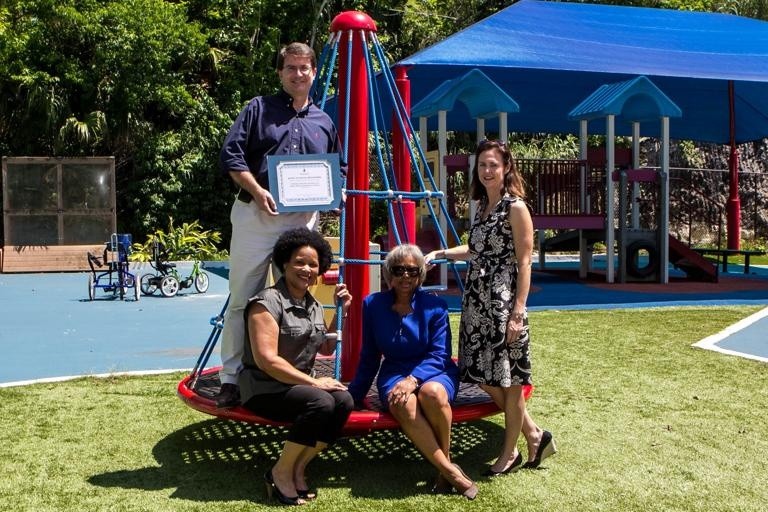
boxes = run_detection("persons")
[349,245,479,500]
[215,42,348,407]
[425,141,556,477]
[238,229,353,505]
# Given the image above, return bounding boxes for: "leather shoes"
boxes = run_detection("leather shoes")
[215,383,240,407]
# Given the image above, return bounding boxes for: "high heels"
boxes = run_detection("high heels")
[481,453,522,476]
[263,468,302,506]
[522,430,557,468]
[296,488,316,499]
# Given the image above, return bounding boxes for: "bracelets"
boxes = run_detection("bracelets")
[442,250,446,259]
[512,311,527,320]
[407,375,419,388]
[336,311,348,318]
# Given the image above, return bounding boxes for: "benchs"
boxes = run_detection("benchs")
[691,245,766,274]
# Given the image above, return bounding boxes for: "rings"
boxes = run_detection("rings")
[393,392,398,396]
[402,390,406,395]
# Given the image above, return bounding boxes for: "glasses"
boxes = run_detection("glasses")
[388,265,420,278]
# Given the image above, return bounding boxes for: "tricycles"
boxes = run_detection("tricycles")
[140,240,209,298]
[87,233,140,301]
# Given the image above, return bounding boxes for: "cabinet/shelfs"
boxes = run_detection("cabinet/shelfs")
[2,155,118,272]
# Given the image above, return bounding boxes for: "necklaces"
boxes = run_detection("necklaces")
[401,306,412,315]
[290,296,306,307]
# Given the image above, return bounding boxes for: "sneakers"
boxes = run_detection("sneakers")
[432,475,453,493]
[452,463,478,500]
